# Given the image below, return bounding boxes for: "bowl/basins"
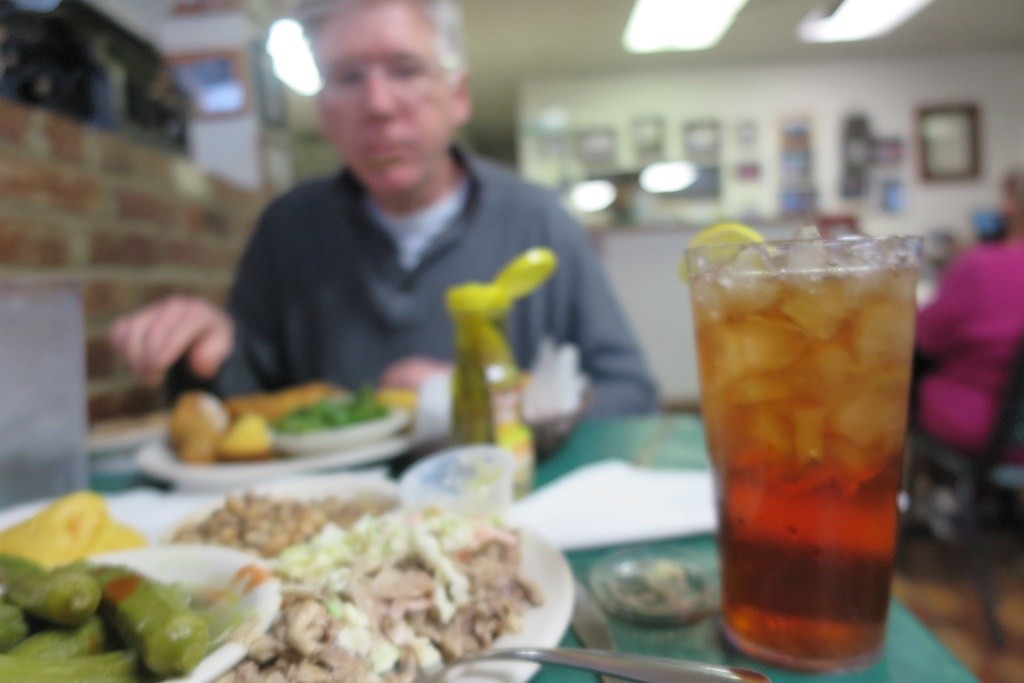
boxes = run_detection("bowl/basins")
[403,443,513,520]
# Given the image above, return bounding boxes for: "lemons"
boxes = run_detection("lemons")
[675,224,779,313]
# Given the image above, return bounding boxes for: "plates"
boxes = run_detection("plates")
[48,547,281,683]
[157,493,574,683]
[135,410,421,481]
[259,411,412,450]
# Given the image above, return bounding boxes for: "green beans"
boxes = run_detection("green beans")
[1,550,208,683]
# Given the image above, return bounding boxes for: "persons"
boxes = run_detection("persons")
[99,0,662,448]
[907,163,1024,536]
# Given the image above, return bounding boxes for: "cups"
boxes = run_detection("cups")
[680,239,915,662]
[0,273,89,497]
